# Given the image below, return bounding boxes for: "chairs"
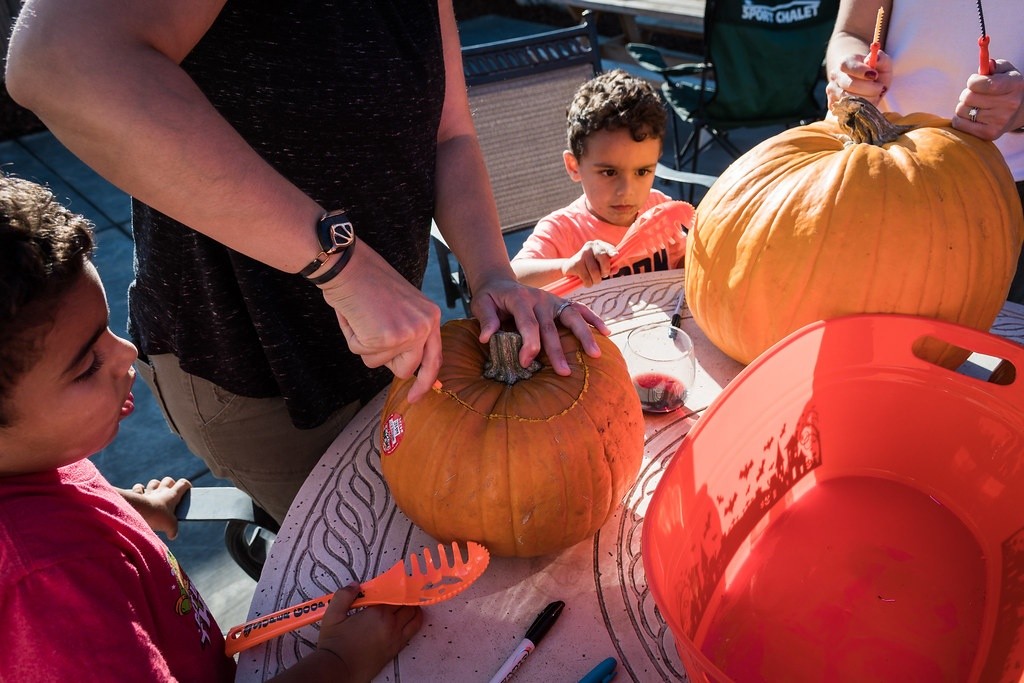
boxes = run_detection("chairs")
[624,0,841,214]
[430,9,605,320]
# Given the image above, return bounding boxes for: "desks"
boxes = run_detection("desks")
[233,268,1024,683]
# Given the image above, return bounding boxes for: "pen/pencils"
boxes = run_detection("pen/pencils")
[667,288,686,340]
[489,600,568,683]
[577,654,624,683]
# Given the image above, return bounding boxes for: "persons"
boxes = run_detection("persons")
[0,179,422,682]
[825,0,1024,303]
[3,0,610,529]
[511,67,688,287]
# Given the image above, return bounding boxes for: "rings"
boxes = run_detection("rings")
[839,90,844,101]
[969,107,979,122]
[555,301,573,317]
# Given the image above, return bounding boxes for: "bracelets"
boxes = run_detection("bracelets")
[301,213,356,282]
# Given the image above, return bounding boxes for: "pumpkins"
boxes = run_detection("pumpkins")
[380,319,643,560]
[684,98,1024,370]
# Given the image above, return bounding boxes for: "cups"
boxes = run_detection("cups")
[624,324,695,414]
